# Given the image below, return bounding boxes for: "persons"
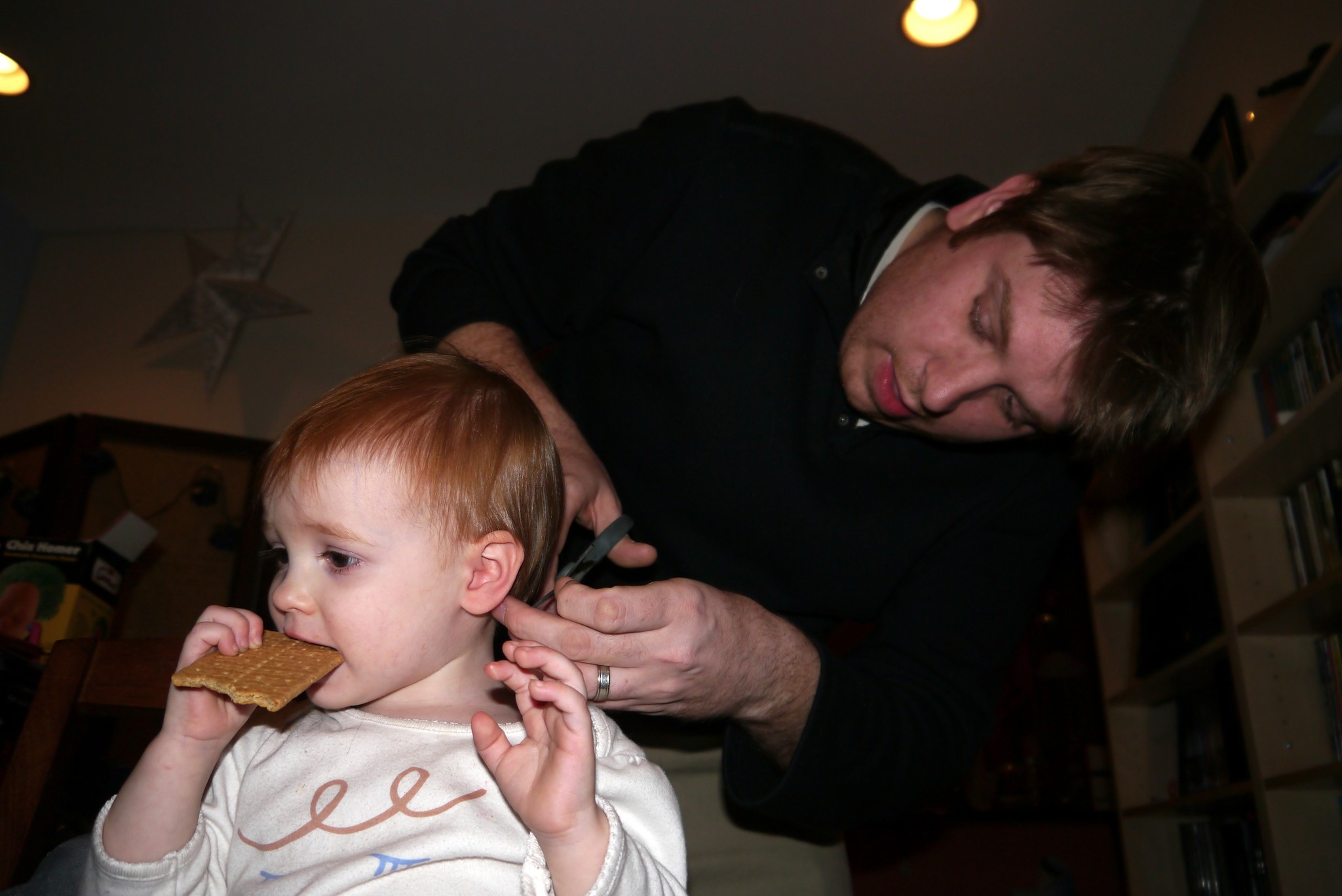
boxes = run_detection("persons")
[385,91,1258,895]
[0,353,687,896]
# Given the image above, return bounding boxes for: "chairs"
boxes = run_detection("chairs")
[0,636,184,890]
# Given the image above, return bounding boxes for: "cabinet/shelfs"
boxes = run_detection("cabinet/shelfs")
[1076,35,1342,896]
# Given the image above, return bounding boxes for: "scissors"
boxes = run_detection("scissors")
[530,514,634,615]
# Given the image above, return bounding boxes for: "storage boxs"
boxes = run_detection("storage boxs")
[0,513,160,666]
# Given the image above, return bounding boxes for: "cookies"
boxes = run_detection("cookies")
[172,630,345,712]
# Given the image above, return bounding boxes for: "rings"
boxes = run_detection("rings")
[593,664,611,702]
